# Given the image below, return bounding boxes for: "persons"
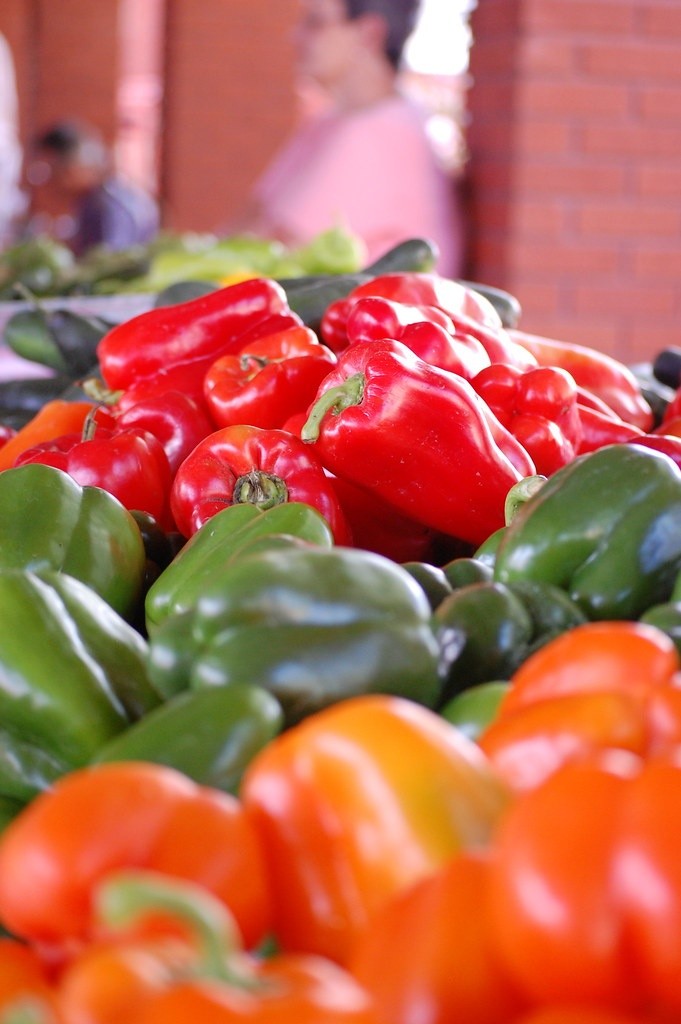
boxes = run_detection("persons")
[235,0,469,281]
[33,117,160,266]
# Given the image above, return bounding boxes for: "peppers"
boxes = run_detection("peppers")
[0,271,681,1024]
